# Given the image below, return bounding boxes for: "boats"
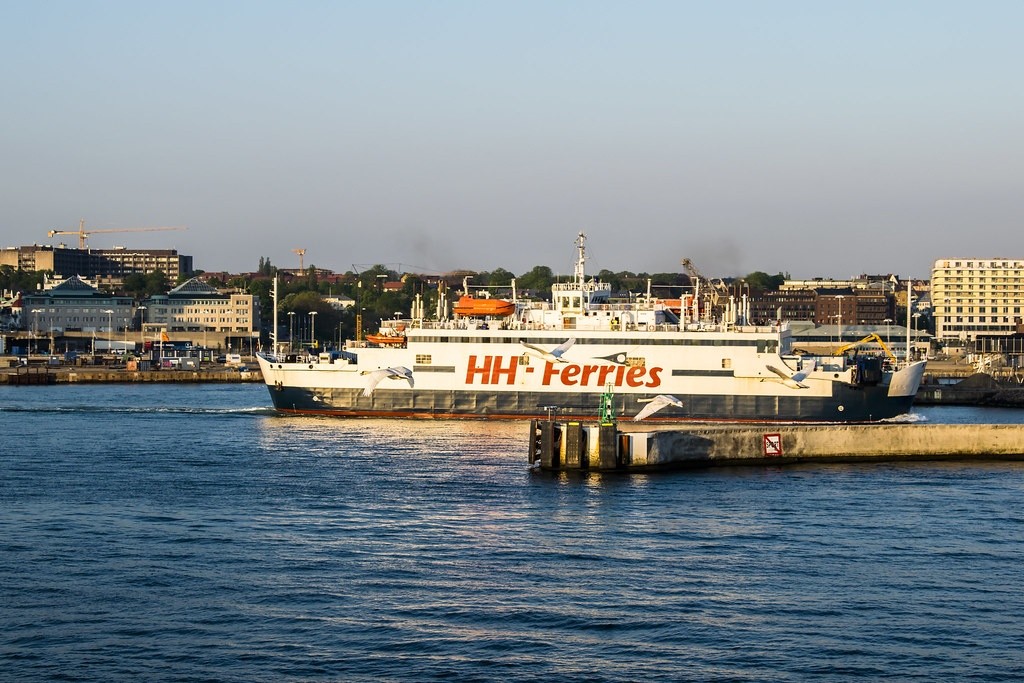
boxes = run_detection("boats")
[364,334,404,343]
[255,229,928,417]
[451,297,516,317]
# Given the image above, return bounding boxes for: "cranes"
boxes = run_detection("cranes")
[48,217,189,248]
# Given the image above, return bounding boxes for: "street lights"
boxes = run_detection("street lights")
[31,309,43,353]
[308,311,317,348]
[827,315,837,355]
[912,312,921,351]
[835,295,844,341]
[340,322,342,351]
[138,306,146,351]
[106,310,114,352]
[882,319,893,348]
[287,312,295,354]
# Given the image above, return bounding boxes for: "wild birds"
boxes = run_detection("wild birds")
[519,338,578,364]
[360,366,415,397]
[631,394,685,423]
[760,360,815,389]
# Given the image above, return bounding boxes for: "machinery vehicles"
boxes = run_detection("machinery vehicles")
[835,333,897,365]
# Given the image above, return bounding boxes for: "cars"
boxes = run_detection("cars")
[891,351,952,361]
[216,355,226,362]
[73,349,84,354]
[226,367,250,372]
